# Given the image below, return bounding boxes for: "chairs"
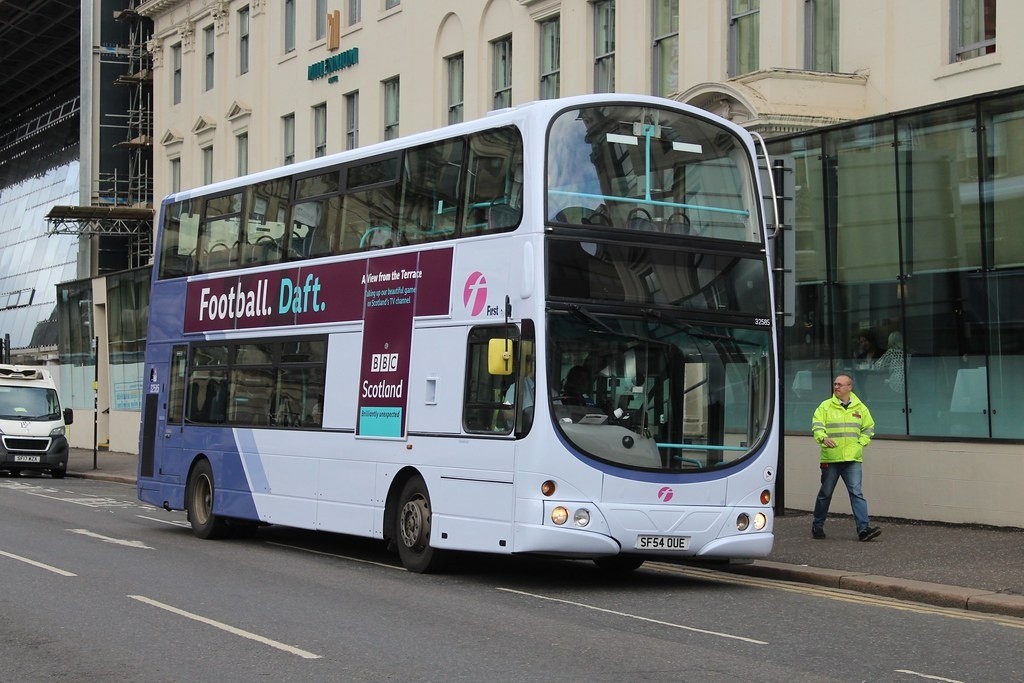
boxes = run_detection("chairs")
[159,194,690,279]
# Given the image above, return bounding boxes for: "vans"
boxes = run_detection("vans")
[0,364,74,478]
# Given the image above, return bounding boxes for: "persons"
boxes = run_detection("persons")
[850,332,886,372]
[869,331,916,398]
[500,358,563,432]
[562,351,610,409]
[811,375,882,542]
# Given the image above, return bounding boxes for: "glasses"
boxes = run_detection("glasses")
[834,383,851,387]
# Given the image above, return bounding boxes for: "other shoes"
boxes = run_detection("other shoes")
[812,527,826,540]
[859,526,882,542]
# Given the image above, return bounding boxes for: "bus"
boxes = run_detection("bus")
[133,92,782,573]
